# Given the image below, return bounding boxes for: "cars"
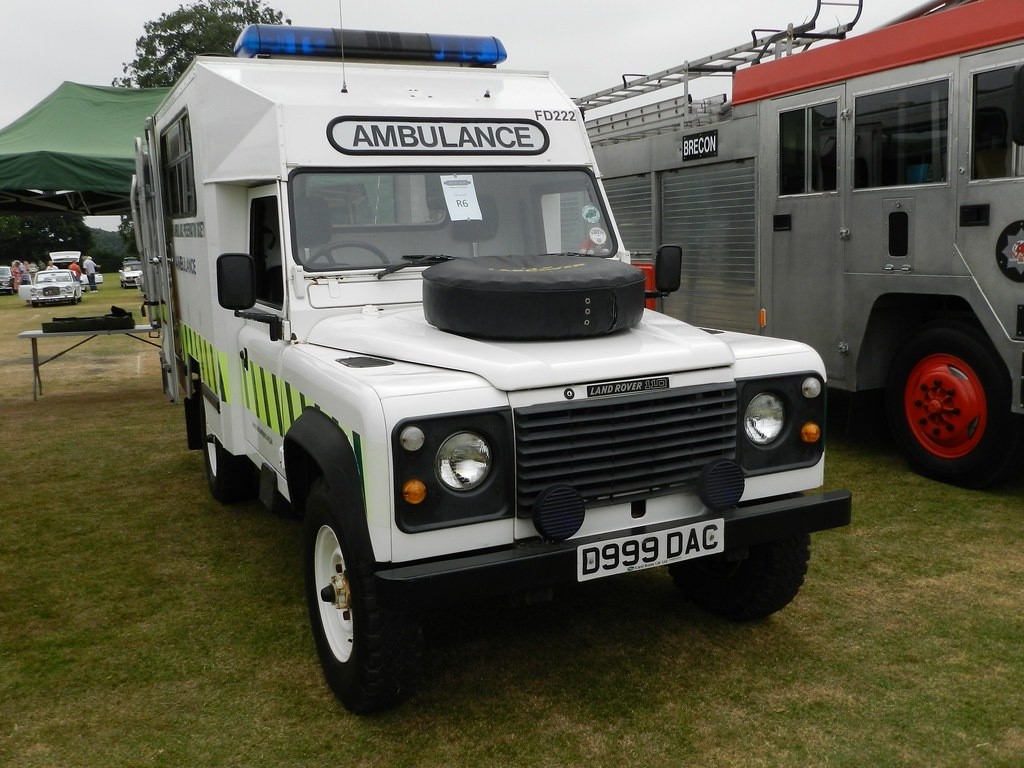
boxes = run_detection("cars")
[48,250,104,286]
[118,256,144,289]
[17,269,83,308]
[0,266,18,295]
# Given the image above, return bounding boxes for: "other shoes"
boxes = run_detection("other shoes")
[90,291,93,293]
[93,290,98,293]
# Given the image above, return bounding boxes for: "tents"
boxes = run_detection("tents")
[0,81,174,215]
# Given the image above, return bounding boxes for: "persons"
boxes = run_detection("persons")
[10,260,46,295]
[46,261,58,270]
[68,260,84,294]
[82,256,101,293]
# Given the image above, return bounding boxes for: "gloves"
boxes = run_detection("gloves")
[86,272,90,276]
[97,265,100,268]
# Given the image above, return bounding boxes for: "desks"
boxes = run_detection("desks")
[17,324,159,401]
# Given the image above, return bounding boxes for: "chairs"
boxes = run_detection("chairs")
[451,194,499,256]
[295,196,335,261]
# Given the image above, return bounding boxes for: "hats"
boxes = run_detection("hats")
[87,256,92,260]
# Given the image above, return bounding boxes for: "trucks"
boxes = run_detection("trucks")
[392,0,1023,493]
[122,21,857,719]
